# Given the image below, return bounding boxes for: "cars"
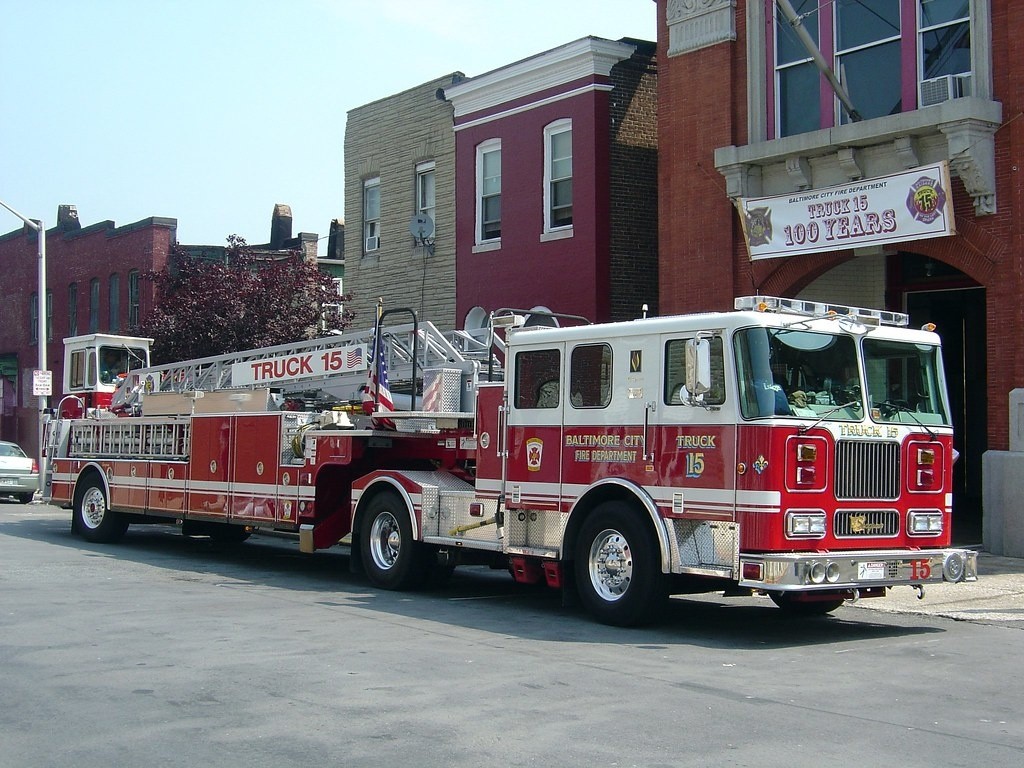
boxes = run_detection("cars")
[0,439,40,505]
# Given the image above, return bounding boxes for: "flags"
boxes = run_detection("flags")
[362,304,398,431]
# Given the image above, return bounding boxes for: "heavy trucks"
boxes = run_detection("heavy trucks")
[30,291,979,630]
